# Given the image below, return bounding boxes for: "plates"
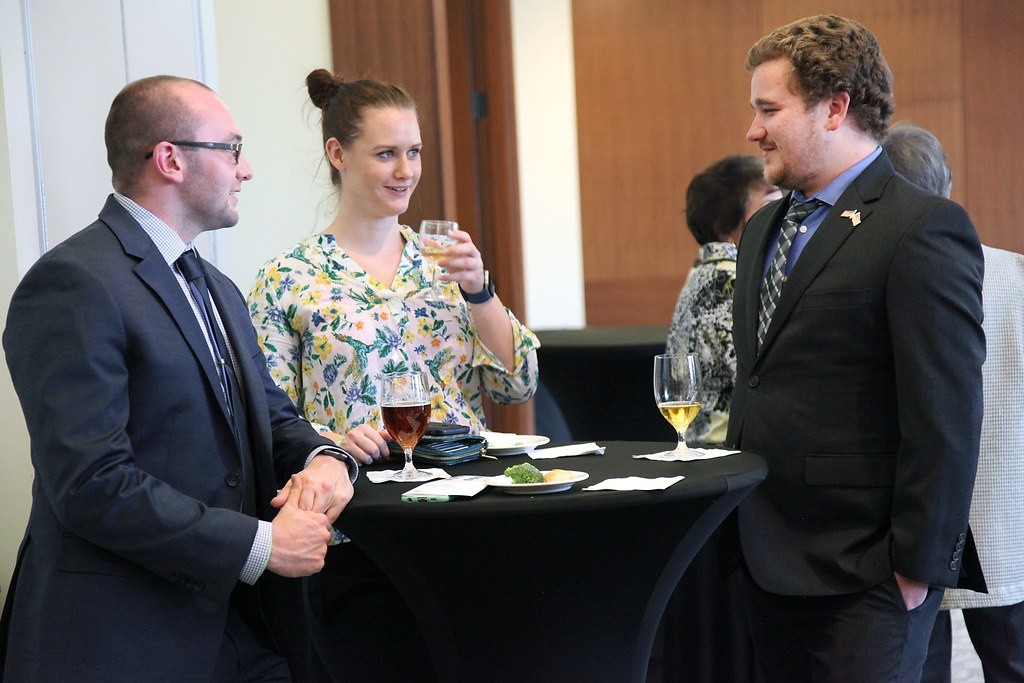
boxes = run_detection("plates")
[481,470,589,494]
[486,435,550,455]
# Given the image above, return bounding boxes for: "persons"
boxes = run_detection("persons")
[724,15,991,682]
[881,121,1023,683]
[663,149,783,446]
[0,75,360,683]
[247,68,542,465]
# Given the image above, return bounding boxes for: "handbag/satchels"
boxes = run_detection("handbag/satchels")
[384,432,498,466]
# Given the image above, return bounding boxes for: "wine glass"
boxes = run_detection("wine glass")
[379,371,432,482]
[652,353,705,460]
[418,219,459,303]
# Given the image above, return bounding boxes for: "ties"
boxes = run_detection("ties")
[757,198,825,355]
[177,250,255,518]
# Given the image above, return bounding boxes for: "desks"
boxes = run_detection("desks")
[244,442,769,683]
[533,326,679,443]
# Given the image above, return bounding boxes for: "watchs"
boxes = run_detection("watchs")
[319,448,349,461]
[458,270,496,305]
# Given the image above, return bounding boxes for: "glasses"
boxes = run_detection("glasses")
[144,141,241,165]
